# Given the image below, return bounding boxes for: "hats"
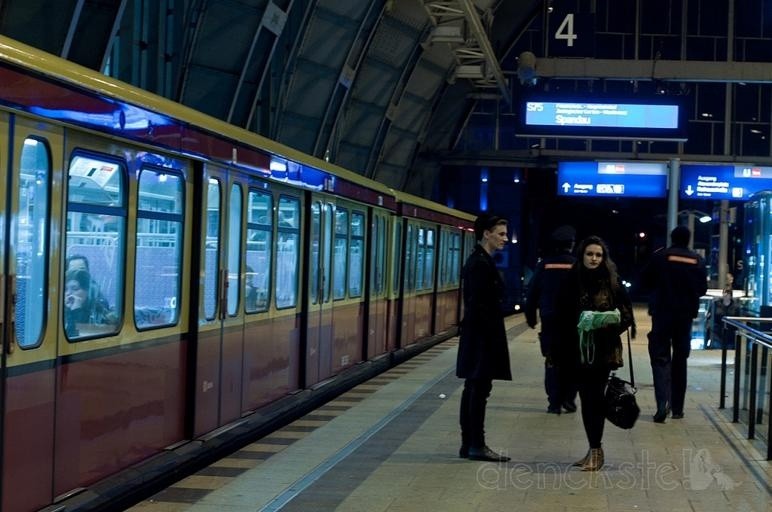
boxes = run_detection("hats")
[474,215,509,241]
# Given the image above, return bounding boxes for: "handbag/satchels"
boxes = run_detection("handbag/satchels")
[577,308,621,364]
[606,376,640,429]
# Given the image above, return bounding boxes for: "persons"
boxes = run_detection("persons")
[454,210,514,463]
[546,236,633,472]
[66,253,111,312]
[61,269,120,338]
[522,226,581,415]
[640,225,708,423]
[704,287,741,351]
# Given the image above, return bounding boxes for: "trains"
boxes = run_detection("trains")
[1,31,478,512]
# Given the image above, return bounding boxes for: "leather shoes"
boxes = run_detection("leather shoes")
[654,409,666,422]
[470,446,511,461]
[673,413,683,419]
[460,446,469,458]
[563,400,576,411]
[573,450,590,466]
[548,401,561,414]
[582,448,603,471]
